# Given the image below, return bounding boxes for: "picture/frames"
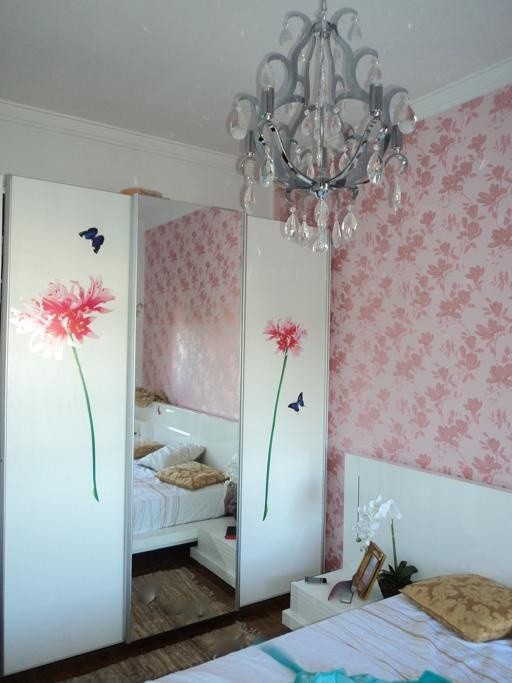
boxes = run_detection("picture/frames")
[353,543,385,599]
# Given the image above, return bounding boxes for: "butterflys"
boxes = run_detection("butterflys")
[288,391,304,412]
[78,228,104,254]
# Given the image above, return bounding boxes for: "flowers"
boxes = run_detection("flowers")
[351,493,417,579]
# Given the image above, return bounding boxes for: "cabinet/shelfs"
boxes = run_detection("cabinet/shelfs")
[240,213,331,622]
[1,172,131,683]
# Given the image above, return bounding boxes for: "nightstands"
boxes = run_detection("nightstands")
[281,569,384,631]
[190,514,236,598]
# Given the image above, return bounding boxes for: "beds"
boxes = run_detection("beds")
[133,388,240,575]
[143,449,512,683]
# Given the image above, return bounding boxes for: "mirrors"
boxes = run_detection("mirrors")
[123,193,248,657]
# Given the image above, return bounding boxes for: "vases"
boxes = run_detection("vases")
[377,573,411,598]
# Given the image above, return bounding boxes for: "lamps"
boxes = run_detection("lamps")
[226,0,419,255]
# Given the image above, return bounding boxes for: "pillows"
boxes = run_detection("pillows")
[398,573,512,643]
[133,436,231,491]
